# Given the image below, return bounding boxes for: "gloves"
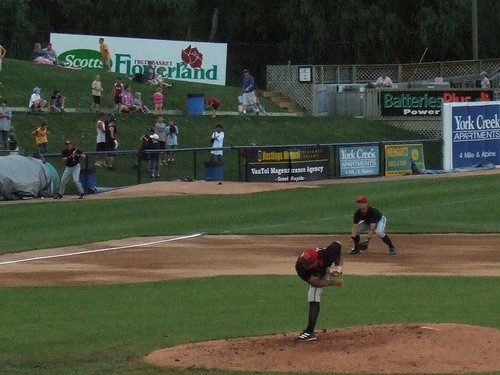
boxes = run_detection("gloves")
[74,153,81,157]
[68,155,73,159]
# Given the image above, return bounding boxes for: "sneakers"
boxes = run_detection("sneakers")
[55,193,63,199]
[297,332,317,341]
[349,249,361,255]
[80,193,87,199]
[389,247,397,255]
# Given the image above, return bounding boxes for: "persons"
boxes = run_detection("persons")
[204,98,220,117]
[90,74,104,112]
[43,43,70,66]
[376,70,392,87]
[479,71,491,88]
[29,87,48,112]
[153,87,164,115]
[104,117,117,170]
[99,38,110,71]
[33,123,50,154]
[153,69,174,87]
[50,90,66,112]
[131,127,157,167]
[7,127,20,155]
[0,46,6,70]
[295,239,343,342]
[141,61,158,86]
[347,195,397,255]
[242,69,259,117]
[165,119,178,163]
[209,124,225,167]
[154,115,166,149]
[149,134,162,179]
[133,93,152,114]
[33,43,58,66]
[94,113,107,167]
[53,139,86,199]
[121,84,141,113]
[0,97,12,150]
[111,77,123,113]
[105,113,117,135]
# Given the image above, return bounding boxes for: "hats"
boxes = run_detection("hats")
[356,196,368,202]
[66,140,71,145]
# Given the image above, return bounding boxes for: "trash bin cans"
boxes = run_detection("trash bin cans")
[204,160,224,182]
[75,167,95,195]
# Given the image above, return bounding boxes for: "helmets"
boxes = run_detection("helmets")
[300,249,317,264]
